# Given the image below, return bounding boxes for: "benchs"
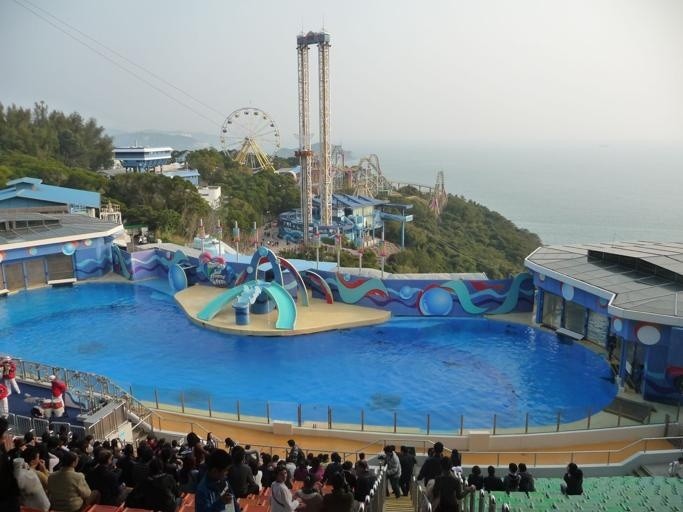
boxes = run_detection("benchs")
[464,474,683,511]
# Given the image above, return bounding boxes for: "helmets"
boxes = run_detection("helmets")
[4,356,11,361]
[48,375,56,381]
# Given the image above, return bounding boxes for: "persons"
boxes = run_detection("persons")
[560,461,583,495]
[0,382,10,420]
[1,414,536,512]
[674,456,682,479]
[633,362,643,393]
[46,374,66,400]
[0,354,22,395]
[606,333,617,359]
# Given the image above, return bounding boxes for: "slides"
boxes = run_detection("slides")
[196,280,297,330]
